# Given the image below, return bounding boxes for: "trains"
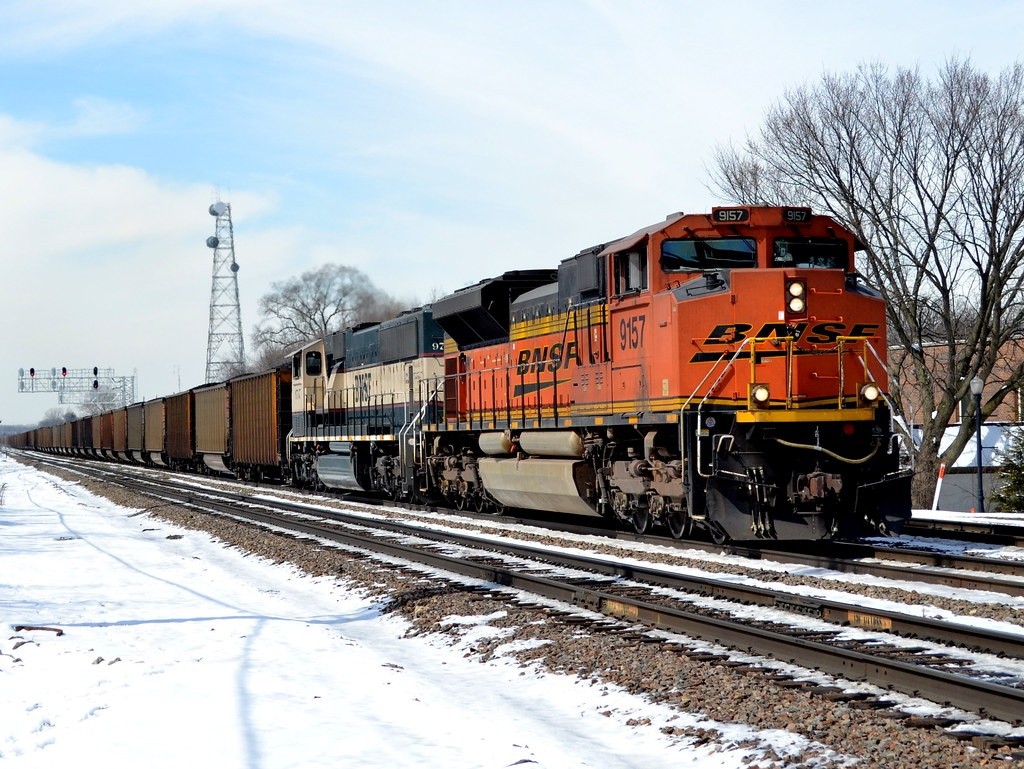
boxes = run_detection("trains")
[0,204,916,544]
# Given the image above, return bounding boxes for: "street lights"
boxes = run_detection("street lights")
[971,374,985,513]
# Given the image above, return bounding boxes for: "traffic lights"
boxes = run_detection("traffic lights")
[94,380,98,389]
[94,367,98,375]
[62,367,66,376]
[30,368,34,376]
